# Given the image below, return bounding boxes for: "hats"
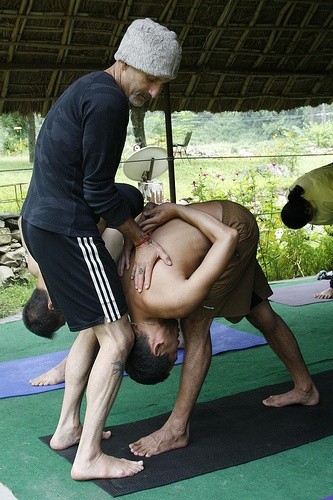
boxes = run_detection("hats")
[114,17,182,79]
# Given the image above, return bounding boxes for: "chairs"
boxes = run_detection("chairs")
[170,130,194,164]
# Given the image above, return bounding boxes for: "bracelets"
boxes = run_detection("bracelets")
[134,236,152,248]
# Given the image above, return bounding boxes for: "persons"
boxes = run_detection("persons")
[19,19,183,481]
[17,184,145,387]
[280,162,333,300]
[116,200,319,457]
